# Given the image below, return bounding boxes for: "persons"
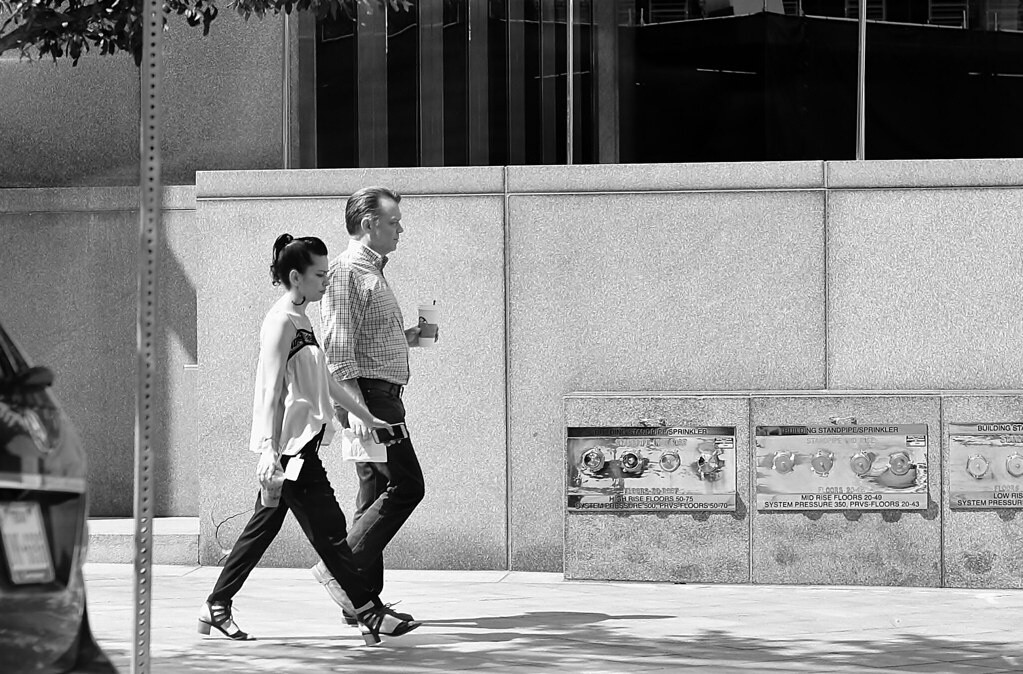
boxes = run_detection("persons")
[312,187,439,627]
[196,233,424,646]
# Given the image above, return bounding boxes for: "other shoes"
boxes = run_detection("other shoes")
[341,604,413,624]
[311,559,354,616]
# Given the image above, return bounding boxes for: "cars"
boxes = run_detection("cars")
[0,319,118,674]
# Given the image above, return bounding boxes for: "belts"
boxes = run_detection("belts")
[357,378,400,397]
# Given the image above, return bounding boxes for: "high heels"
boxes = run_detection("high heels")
[355,605,422,646]
[197,601,256,640]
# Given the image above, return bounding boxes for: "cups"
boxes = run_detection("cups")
[416,304,440,347]
[260,471,287,507]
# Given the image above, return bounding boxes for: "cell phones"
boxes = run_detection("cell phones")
[371,422,409,444]
